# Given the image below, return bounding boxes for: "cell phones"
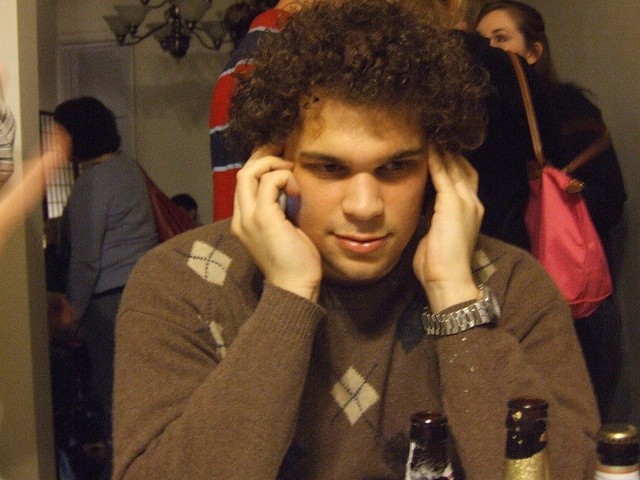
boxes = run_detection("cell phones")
[277,189,295,219]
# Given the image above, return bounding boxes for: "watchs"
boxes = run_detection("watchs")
[420,284,502,337]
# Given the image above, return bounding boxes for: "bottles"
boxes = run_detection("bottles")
[595,422,640,480]
[406,410,454,479]
[502,395,550,480]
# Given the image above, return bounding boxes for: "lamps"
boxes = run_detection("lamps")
[102,1,241,60]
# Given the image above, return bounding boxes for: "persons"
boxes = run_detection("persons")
[404,0,553,248]
[167,194,203,228]
[210,1,317,220]
[476,0,626,422]
[113,0,601,479]
[38,98,160,479]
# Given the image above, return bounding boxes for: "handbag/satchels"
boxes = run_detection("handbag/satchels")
[142,167,197,243]
[522,159,613,319]
